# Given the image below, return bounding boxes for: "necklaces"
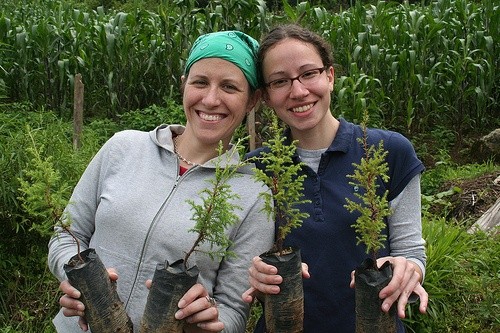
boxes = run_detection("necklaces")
[175,134,203,167]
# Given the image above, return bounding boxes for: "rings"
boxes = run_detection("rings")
[205,295,216,307]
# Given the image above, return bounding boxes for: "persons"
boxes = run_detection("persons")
[47,30,276,333]
[241,25,429,333]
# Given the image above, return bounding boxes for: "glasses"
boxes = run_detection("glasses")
[263,66,327,93]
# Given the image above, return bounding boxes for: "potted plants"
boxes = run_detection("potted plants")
[251,109,304,333]
[17,125,135,333]
[137,135,251,333]
[352,109,398,333]
